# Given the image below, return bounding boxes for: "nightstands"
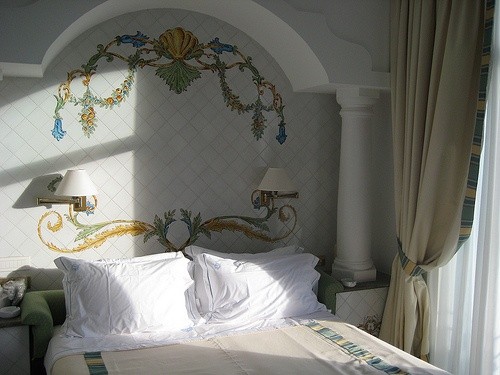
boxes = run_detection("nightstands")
[335,275,392,337]
[0,319,31,375]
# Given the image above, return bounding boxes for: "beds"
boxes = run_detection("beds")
[22,267,452,375]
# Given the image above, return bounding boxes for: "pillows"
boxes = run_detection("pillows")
[63,259,192,331]
[186,245,305,277]
[197,252,324,324]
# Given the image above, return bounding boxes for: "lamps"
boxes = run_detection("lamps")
[259,167,299,207]
[36,169,100,211]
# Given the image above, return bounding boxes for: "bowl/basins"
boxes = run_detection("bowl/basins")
[341,278,356,287]
[0,306,20,318]
